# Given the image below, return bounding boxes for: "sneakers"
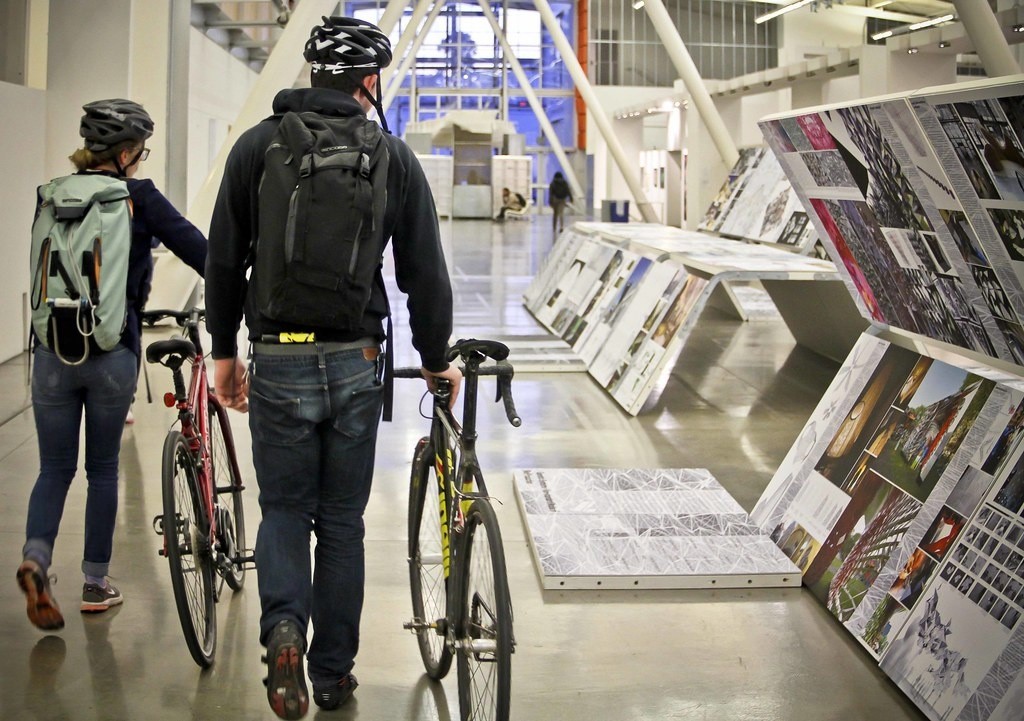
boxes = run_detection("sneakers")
[25,637,66,710]
[314,673,359,709]
[79,583,122,611]
[17,562,63,629]
[261,618,309,720]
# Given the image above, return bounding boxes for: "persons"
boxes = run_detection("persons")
[548,171,573,232]
[206,18,463,721]
[15,97,249,632]
[497,188,523,220]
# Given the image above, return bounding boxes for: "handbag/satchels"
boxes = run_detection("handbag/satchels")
[247,112,387,330]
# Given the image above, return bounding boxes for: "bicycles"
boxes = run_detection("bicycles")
[391,339,522,721]
[135,310,257,667]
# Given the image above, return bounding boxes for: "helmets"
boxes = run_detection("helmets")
[303,15,393,75]
[80,99,154,152]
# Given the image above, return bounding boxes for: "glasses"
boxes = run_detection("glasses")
[121,145,150,162]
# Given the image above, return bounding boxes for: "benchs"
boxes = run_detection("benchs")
[754,0,814,24]
[505,198,536,223]
[872,14,955,40]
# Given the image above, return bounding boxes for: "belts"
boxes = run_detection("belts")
[254,337,376,358]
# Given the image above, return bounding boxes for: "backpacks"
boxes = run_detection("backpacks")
[30,174,137,355]
